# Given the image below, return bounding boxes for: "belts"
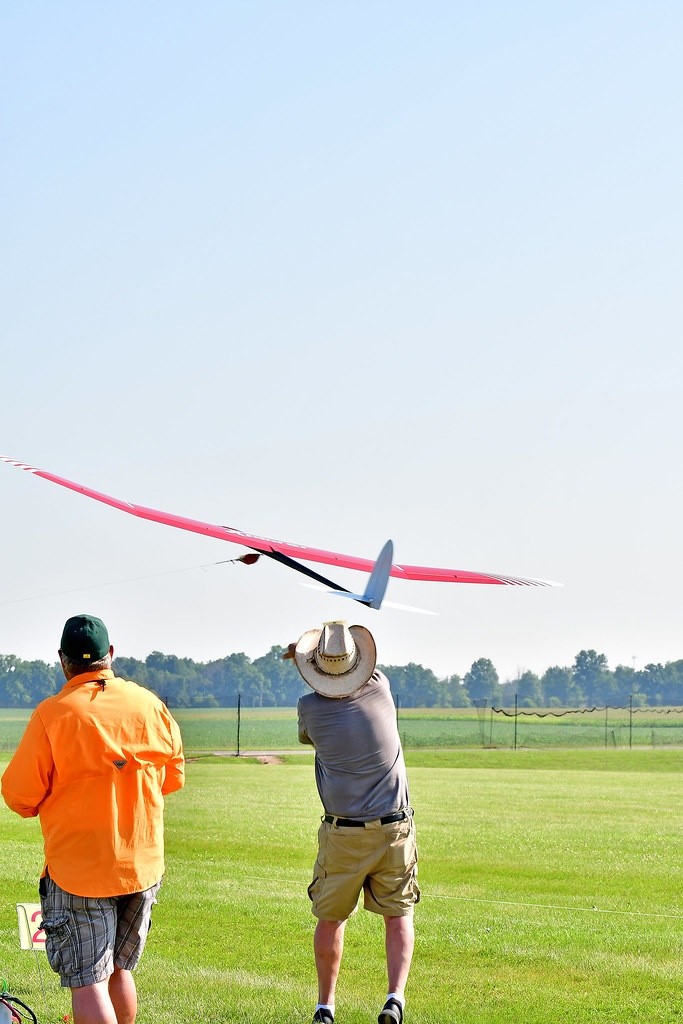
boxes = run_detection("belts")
[325,810,413,827]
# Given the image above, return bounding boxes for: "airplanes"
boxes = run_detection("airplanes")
[0,453,567,613]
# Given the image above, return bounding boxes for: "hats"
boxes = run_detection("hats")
[61,614,109,666]
[293,621,376,699]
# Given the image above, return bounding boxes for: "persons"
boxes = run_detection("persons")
[284,622,421,1024]
[1,614,186,1024]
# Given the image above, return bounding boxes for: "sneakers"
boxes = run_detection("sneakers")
[377,997,403,1024]
[312,1008,333,1024]
